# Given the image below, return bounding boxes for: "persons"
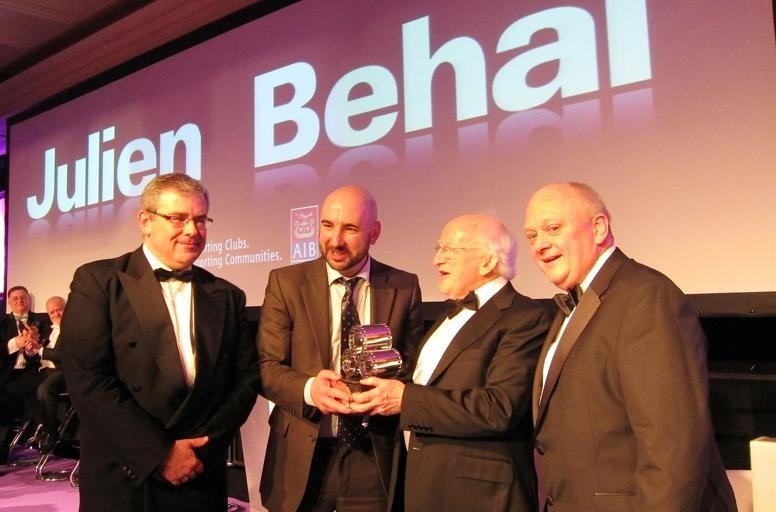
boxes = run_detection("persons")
[254,183,425,511]
[522,182,740,512]
[6,296,67,455]
[0,285,51,470]
[348,213,552,512]
[58,171,262,512]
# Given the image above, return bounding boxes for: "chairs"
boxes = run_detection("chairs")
[8,391,80,489]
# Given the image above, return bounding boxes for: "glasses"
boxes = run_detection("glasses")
[433,243,480,256]
[145,209,213,227]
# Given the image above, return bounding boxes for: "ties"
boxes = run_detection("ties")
[333,278,366,451]
[19,319,27,333]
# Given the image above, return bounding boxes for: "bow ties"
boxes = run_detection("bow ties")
[155,268,194,283]
[553,283,582,316]
[444,291,480,319]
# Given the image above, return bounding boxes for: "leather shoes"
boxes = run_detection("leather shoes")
[25,423,58,454]
